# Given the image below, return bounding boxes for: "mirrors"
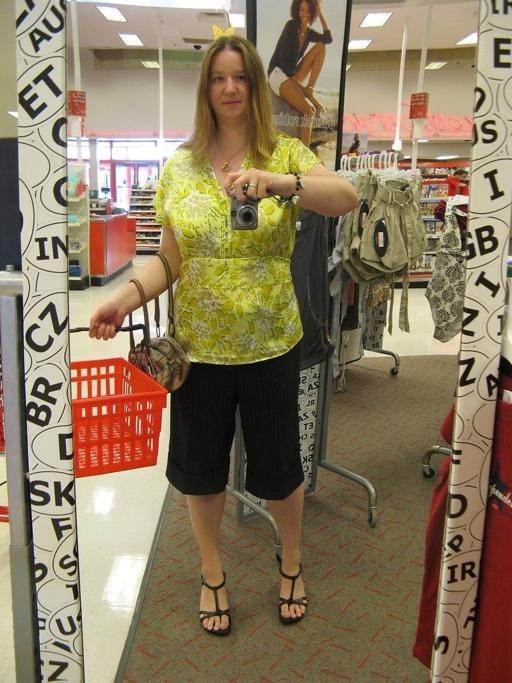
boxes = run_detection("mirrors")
[14,0,512,682]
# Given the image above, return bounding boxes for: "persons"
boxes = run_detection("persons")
[446,169,469,196]
[88,35,360,635]
[267,1,332,148]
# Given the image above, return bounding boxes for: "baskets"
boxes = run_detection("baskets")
[70,357,169,478]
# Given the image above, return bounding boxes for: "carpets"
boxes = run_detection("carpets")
[113,354,458,683]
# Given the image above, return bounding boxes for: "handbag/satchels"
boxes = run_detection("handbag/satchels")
[128,337,192,394]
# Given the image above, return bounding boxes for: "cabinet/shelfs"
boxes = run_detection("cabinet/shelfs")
[125,184,163,253]
[373,160,469,288]
[68,162,92,291]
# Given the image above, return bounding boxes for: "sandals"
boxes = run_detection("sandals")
[275,553,309,626]
[199,571,231,637]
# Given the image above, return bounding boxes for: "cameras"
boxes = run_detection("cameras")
[230,189,258,230]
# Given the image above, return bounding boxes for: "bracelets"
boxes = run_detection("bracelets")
[280,171,304,205]
